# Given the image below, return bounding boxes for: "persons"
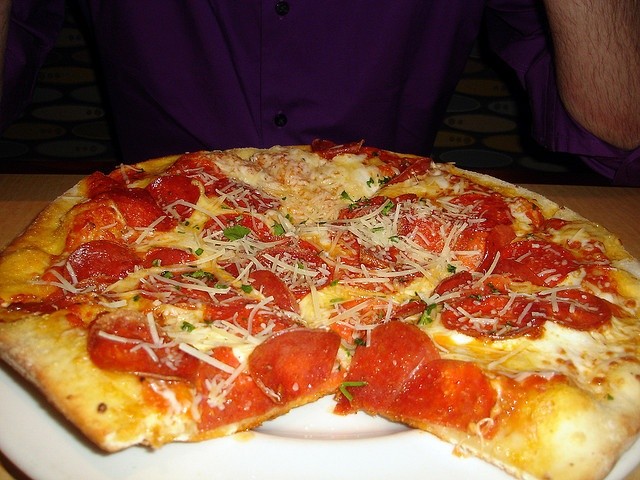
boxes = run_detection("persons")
[0,0,640,186]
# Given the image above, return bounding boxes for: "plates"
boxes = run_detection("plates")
[0,360,640,476]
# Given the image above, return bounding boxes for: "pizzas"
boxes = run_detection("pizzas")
[0,136,640,477]
